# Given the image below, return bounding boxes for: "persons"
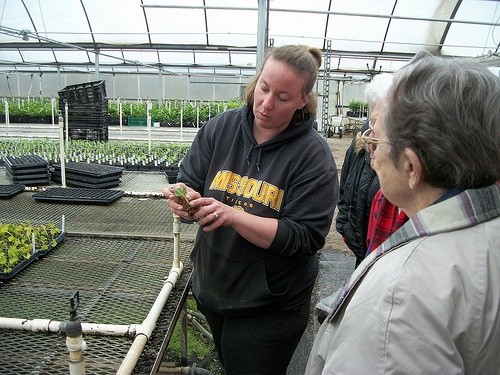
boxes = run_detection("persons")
[334,71,410,277]
[162,43,340,375]
[301,46,500,375]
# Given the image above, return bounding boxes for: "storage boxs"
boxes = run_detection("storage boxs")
[58,80,108,141]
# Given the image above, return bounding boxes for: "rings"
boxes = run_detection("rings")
[213,212,219,220]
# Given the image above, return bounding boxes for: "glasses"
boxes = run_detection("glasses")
[361,128,400,149]
